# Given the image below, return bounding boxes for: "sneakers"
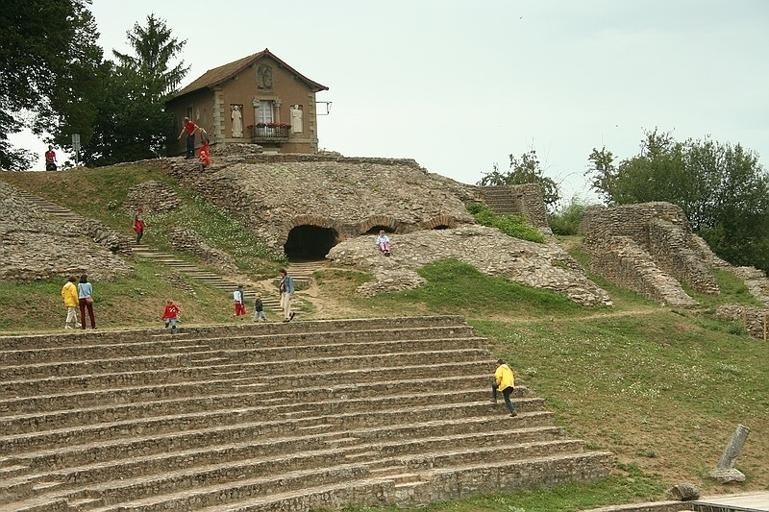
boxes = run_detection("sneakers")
[64,325,72,330]
[489,399,497,403]
[283,320,290,323]
[74,323,82,328]
[290,312,296,320]
[510,412,518,417]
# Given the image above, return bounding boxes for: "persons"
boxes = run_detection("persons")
[197,127,210,167]
[133,208,145,245]
[161,299,180,328]
[45,145,57,171]
[278,269,296,323]
[61,276,82,330]
[291,104,303,133]
[233,283,246,321]
[376,229,390,257]
[176,116,199,160]
[494,359,517,416]
[231,105,243,138]
[253,291,266,323]
[77,275,96,330]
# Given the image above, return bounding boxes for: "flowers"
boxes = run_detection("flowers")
[240,121,292,136]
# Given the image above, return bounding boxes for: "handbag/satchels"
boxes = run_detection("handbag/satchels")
[85,296,94,305]
